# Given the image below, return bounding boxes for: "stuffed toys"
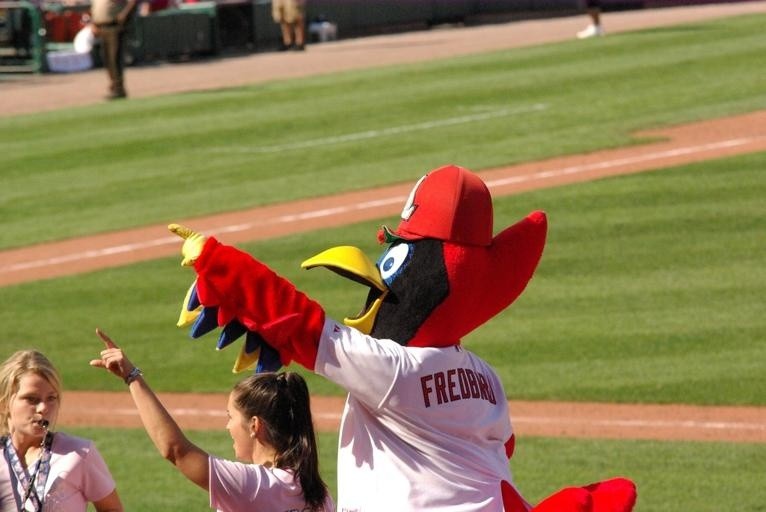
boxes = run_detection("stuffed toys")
[164,164,641,512]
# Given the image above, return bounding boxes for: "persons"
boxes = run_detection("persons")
[0,348,125,511]
[574,1,603,40]
[270,1,308,52]
[87,328,339,511]
[84,0,136,100]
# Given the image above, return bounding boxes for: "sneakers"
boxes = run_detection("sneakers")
[576,26,603,39]
[277,42,306,51]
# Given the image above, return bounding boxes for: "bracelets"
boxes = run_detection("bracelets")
[121,367,144,385]
